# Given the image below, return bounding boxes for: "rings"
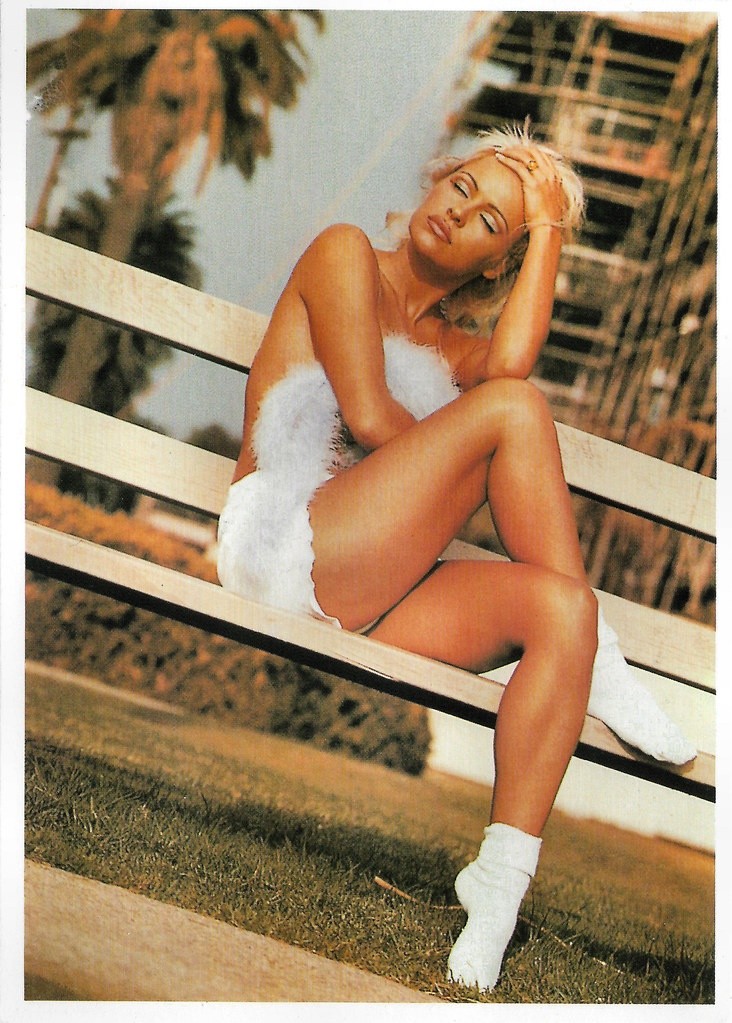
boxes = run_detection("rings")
[526,160,539,175]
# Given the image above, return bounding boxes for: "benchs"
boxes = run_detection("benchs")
[26,228,715,800]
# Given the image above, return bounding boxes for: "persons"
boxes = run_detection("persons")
[217,115,698,993]
